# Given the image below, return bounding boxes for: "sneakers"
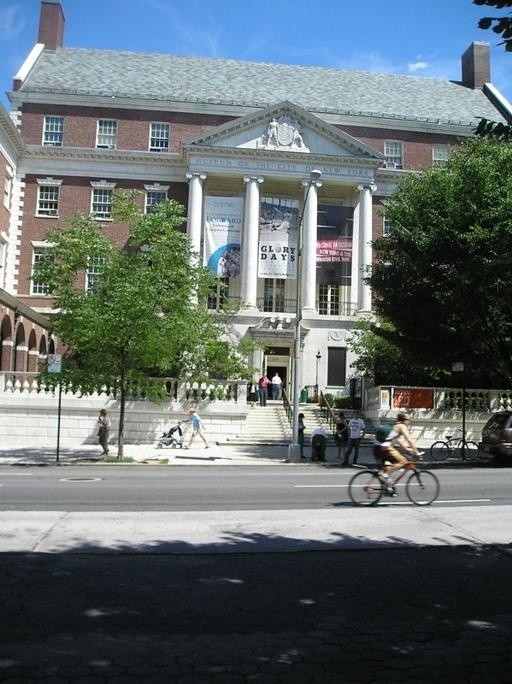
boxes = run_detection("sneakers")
[377,470,394,487]
[383,488,400,497]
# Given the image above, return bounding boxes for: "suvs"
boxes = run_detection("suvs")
[477,409,511,460]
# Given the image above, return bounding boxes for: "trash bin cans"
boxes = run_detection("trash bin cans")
[301,389,308,404]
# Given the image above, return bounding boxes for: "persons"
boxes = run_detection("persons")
[310,428,329,462]
[342,410,368,465]
[372,410,422,497]
[335,411,349,460]
[297,413,308,458]
[181,407,211,450]
[270,370,285,400]
[97,408,112,456]
[258,372,272,406]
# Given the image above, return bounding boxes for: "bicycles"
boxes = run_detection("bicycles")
[347,449,441,506]
[430,428,479,461]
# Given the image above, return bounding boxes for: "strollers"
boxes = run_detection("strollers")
[157,423,184,448]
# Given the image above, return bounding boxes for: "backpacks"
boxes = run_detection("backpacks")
[374,418,394,443]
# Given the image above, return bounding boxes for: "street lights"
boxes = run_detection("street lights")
[287,167,323,461]
[451,350,468,462]
